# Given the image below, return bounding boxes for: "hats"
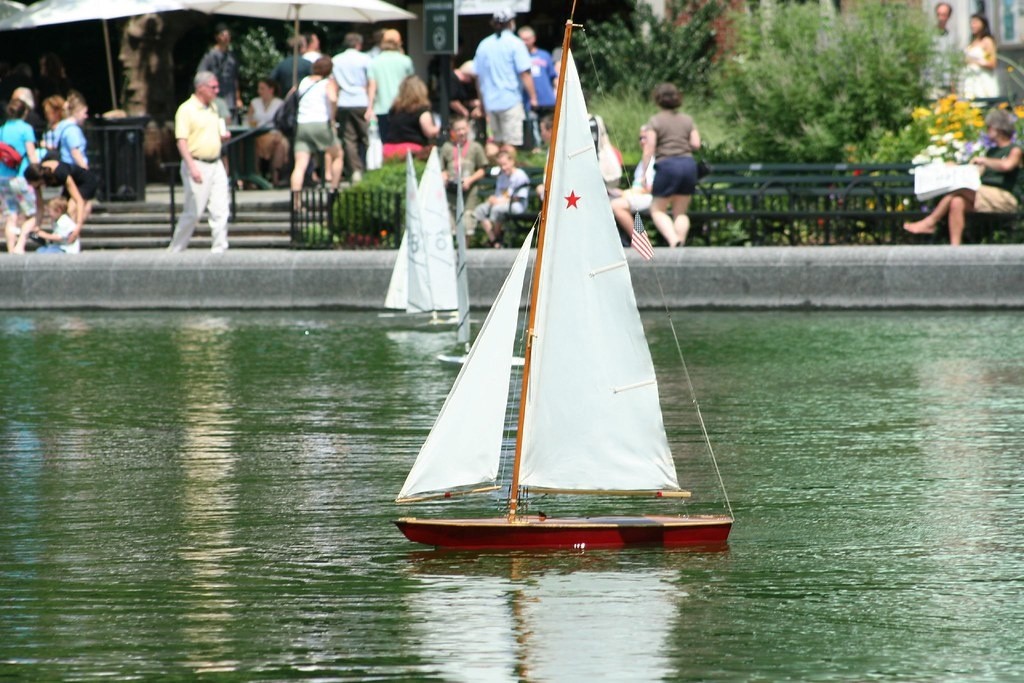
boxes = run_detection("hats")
[493,6,516,23]
[12,87,34,109]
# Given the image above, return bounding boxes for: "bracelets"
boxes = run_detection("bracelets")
[34,229,41,234]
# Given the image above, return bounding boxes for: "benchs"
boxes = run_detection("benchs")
[467,161,1024,244]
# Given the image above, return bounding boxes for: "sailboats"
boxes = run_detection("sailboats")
[380,145,479,333]
[391,0,742,551]
[435,143,526,370]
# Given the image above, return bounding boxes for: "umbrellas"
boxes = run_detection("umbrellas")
[0,0,417,112]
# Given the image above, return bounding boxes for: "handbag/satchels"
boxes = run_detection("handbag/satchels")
[0,142,22,168]
[696,149,710,179]
[274,92,298,141]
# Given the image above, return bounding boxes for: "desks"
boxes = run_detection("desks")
[228,126,276,189]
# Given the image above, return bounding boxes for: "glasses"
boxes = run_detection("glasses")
[203,83,219,90]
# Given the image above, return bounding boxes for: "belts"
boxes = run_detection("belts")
[193,156,218,164]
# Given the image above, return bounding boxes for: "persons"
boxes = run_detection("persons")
[473,7,538,148]
[610,83,702,247]
[247,26,623,248]
[197,22,243,122]
[165,71,233,251]
[904,106,1024,246]
[472,146,531,249]
[959,15,1013,114]
[0,87,98,256]
[920,3,952,100]
[32,198,81,255]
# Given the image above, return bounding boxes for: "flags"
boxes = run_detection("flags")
[632,214,655,262]
